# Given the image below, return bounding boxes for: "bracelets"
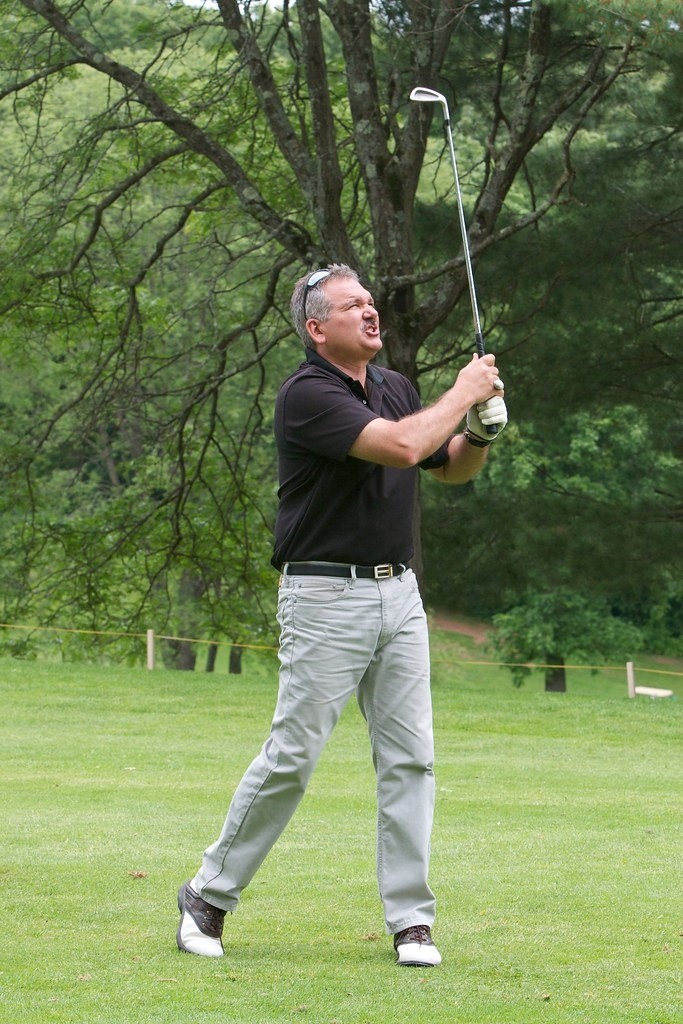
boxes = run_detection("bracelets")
[464,427,491,449]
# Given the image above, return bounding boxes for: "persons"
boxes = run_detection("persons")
[177,266,508,966]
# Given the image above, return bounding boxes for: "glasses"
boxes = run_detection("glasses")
[302,266,331,320]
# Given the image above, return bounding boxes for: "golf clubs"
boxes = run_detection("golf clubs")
[409,85,500,436]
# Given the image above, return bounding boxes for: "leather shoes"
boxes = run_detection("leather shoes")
[394,926,443,966]
[177,881,227,959]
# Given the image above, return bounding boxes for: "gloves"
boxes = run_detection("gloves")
[466,380,508,442]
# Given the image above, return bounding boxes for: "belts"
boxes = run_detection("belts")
[280,562,410,579]
